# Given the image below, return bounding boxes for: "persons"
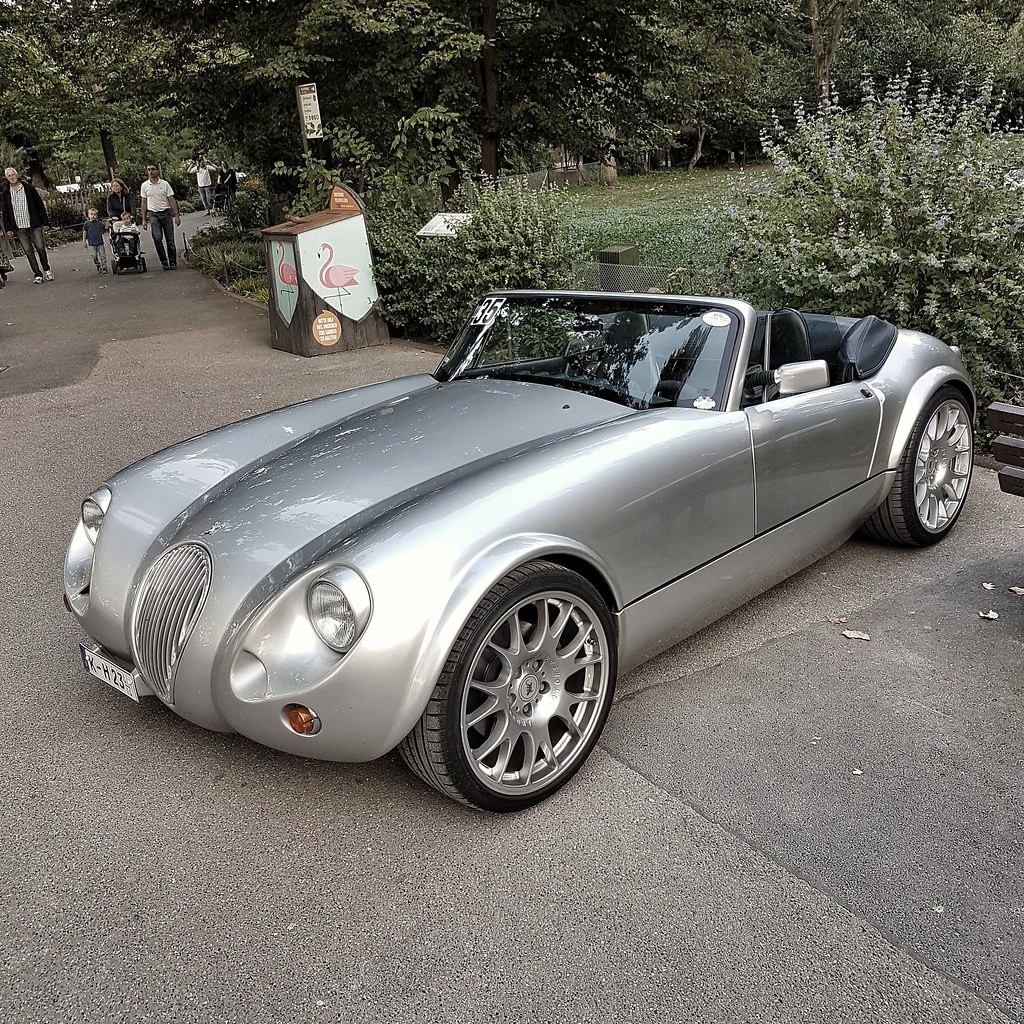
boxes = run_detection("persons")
[0,166,55,284]
[196,155,207,172]
[106,178,137,225]
[188,158,217,217]
[140,162,182,270]
[115,211,140,255]
[218,160,238,201]
[82,208,109,274]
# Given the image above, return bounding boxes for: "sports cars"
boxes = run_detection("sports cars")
[59,284,978,821]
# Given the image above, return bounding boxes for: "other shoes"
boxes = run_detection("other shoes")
[204,209,213,215]
[97,266,107,274]
[33,276,44,284]
[44,270,55,280]
[170,263,177,269]
[162,260,169,270]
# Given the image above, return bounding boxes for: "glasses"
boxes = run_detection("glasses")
[148,168,158,171]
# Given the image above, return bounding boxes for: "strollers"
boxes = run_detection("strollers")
[209,183,231,216]
[108,215,147,276]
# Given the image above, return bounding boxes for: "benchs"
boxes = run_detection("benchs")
[985,401,1024,498]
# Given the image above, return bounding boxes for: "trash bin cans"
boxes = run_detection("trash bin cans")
[259,181,391,357]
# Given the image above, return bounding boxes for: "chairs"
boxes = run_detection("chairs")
[590,308,662,403]
[739,306,815,409]
